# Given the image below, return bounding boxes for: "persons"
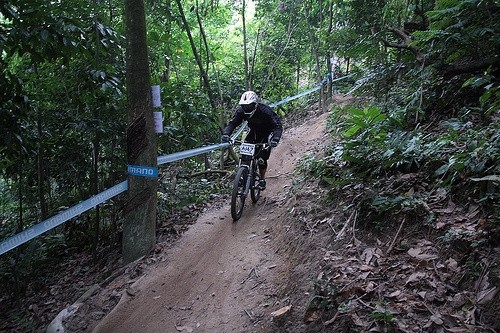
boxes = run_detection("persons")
[221,91,283,189]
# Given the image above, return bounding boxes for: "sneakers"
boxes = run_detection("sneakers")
[258,179,266,190]
[238,177,244,186]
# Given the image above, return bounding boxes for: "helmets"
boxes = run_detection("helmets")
[239,91,259,119]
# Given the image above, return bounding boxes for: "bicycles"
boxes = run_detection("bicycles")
[224,138,274,221]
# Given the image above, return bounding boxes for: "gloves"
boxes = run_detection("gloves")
[221,135,229,141]
[271,139,277,147]
